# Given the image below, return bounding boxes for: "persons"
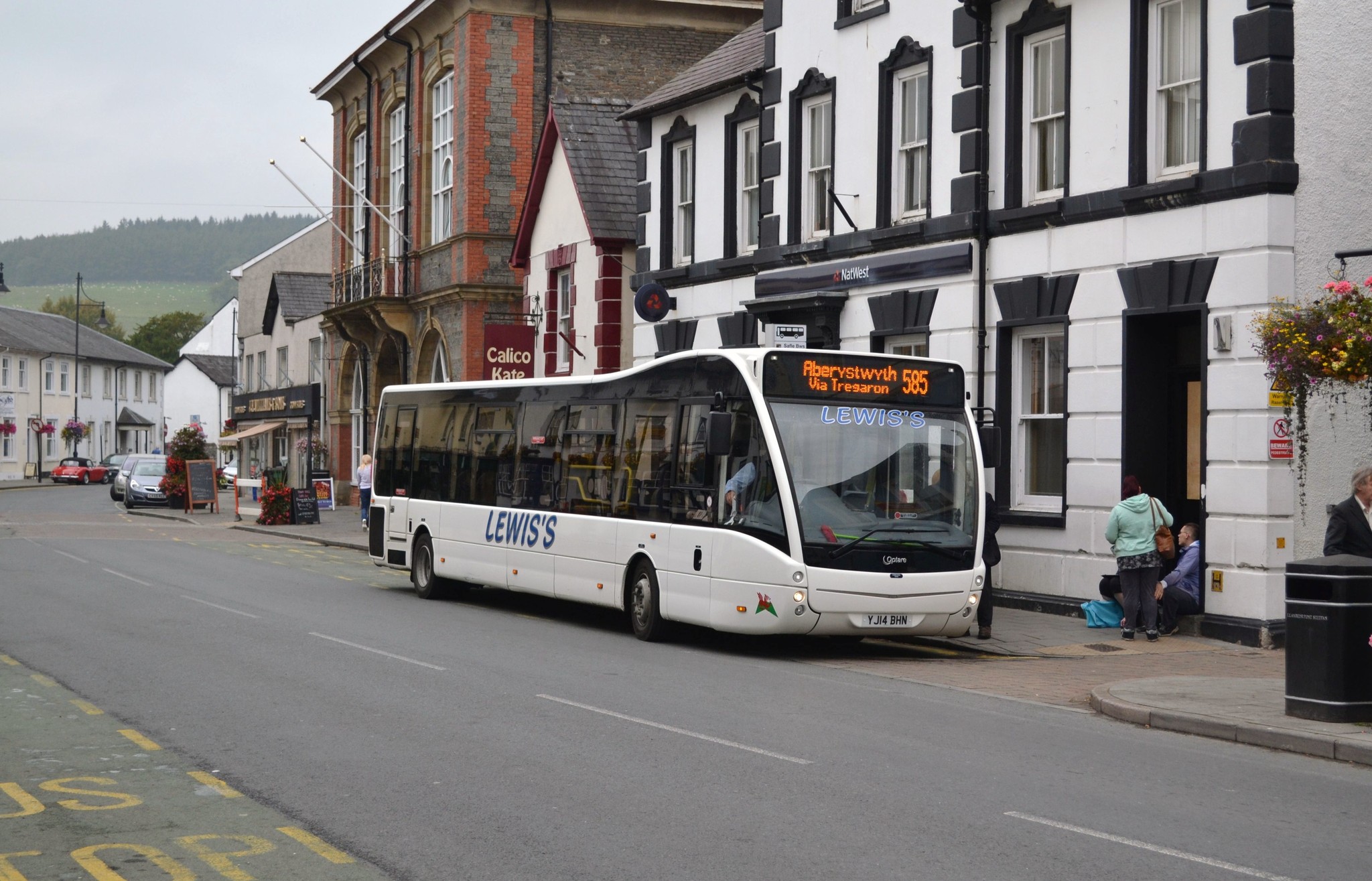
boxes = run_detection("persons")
[724,437,796,518]
[152,447,162,455]
[898,467,1000,640]
[1136,523,1200,637]
[1099,568,1127,628]
[1322,464,1372,559]
[357,455,372,532]
[1105,476,1173,642]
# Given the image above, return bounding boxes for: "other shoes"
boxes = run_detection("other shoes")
[1157,624,1179,636]
[362,519,367,532]
[978,626,991,639]
[947,630,970,638]
[1145,629,1159,642]
[1136,623,1160,633]
[1121,628,1135,641]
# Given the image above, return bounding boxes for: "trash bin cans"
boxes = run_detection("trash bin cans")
[1281,551,1372,723]
[311,469,330,479]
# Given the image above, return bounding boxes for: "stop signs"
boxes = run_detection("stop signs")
[163,425,168,436]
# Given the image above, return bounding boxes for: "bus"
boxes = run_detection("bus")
[368,348,1004,645]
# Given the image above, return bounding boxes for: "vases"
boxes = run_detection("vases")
[165,496,207,509]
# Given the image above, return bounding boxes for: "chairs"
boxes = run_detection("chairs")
[377,439,880,529]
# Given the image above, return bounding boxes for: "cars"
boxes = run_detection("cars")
[110,453,167,501]
[97,454,128,482]
[121,459,168,509]
[221,458,238,483]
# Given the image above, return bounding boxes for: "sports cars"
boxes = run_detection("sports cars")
[49,457,109,485]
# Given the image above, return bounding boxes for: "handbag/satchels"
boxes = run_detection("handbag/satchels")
[1081,599,1124,628]
[1154,525,1176,561]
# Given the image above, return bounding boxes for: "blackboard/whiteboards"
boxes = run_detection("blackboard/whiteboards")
[184,460,218,504]
[290,487,320,523]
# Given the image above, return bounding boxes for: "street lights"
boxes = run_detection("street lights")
[74,272,108,459]
[164,417,172,454]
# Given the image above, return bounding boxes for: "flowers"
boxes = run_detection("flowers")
[0,422,17,434]
[255,467,295,526]
[1245,275,1372,528]
[217,419,240,454]
[158,424,210,496]
[295,434,328,469]
[36,423,57,435]
[60,420,92,452]
[215,468,227,490]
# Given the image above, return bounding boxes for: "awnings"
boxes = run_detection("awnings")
[217,421,286,449]
